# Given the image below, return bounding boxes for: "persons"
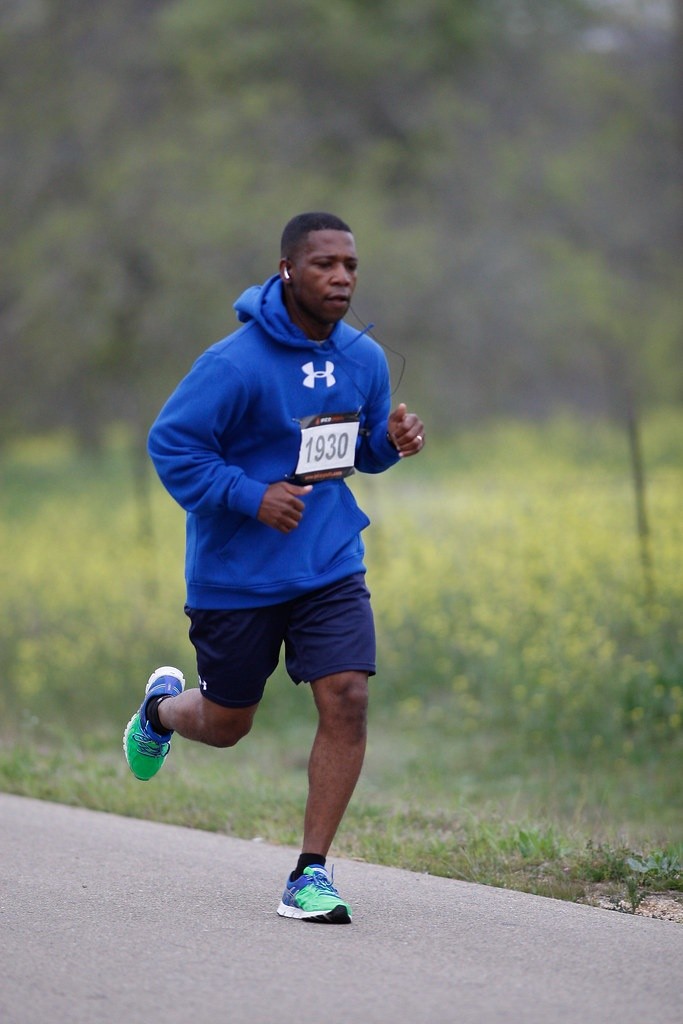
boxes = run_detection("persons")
[121,213,424,925]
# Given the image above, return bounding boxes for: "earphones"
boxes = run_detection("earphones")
[283,266,290,280]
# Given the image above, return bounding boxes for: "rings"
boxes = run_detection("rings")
[417,436,422,441]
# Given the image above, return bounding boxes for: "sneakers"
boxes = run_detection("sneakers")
[277,864,353,924]
[122,666,186,782]
